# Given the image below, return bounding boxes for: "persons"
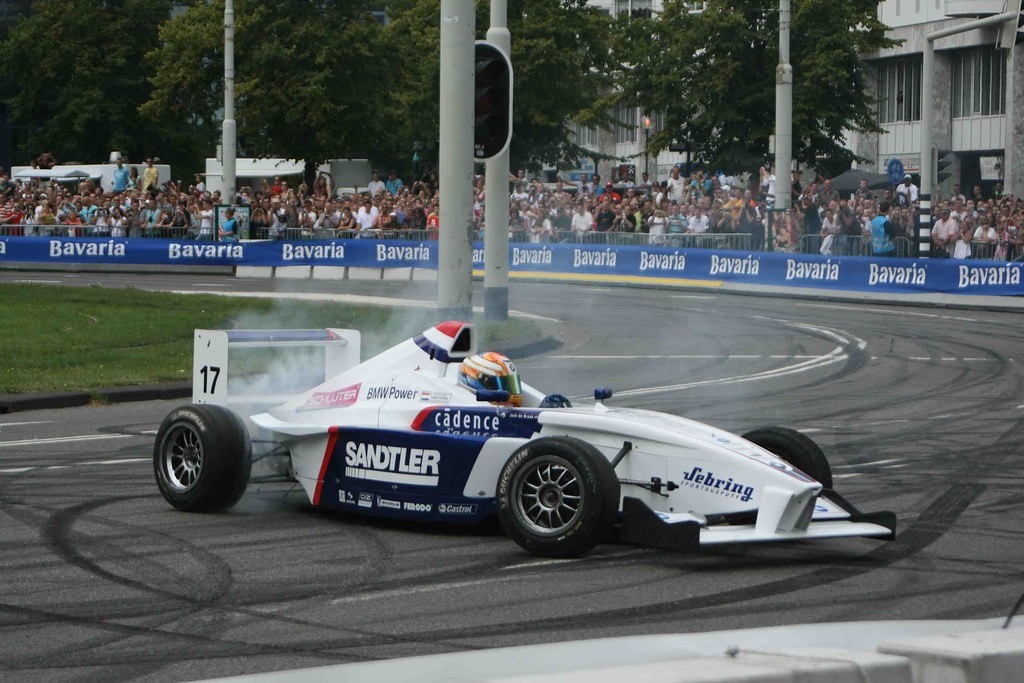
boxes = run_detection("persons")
[219,207,239,242]
[235,170,439,240]
[472,166,920,258]
[460,351,523,407]
[930,183,1024,262]
[31,152,55,169]
[0,157,223,242]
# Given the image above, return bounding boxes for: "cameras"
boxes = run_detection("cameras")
[840,205,846,214]
[725,211,730,215]
[896,212,901,217]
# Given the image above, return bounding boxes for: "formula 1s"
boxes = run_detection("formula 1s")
[152,319,898,560]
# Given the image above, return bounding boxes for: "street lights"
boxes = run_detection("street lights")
[643,116,653,174]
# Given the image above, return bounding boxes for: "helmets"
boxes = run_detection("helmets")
[459,350,523,406]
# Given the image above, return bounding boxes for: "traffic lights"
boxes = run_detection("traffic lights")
[930,147,954,185]
[474,39,514,164]
[1000,1,1024,51]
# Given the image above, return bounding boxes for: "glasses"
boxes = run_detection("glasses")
[981,223,989,226]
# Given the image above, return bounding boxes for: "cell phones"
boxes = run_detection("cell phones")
[622,210,625,215]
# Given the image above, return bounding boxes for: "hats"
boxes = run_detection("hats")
[880,201,891,212]
[941,207,950,213]
[904,174,912,180]
